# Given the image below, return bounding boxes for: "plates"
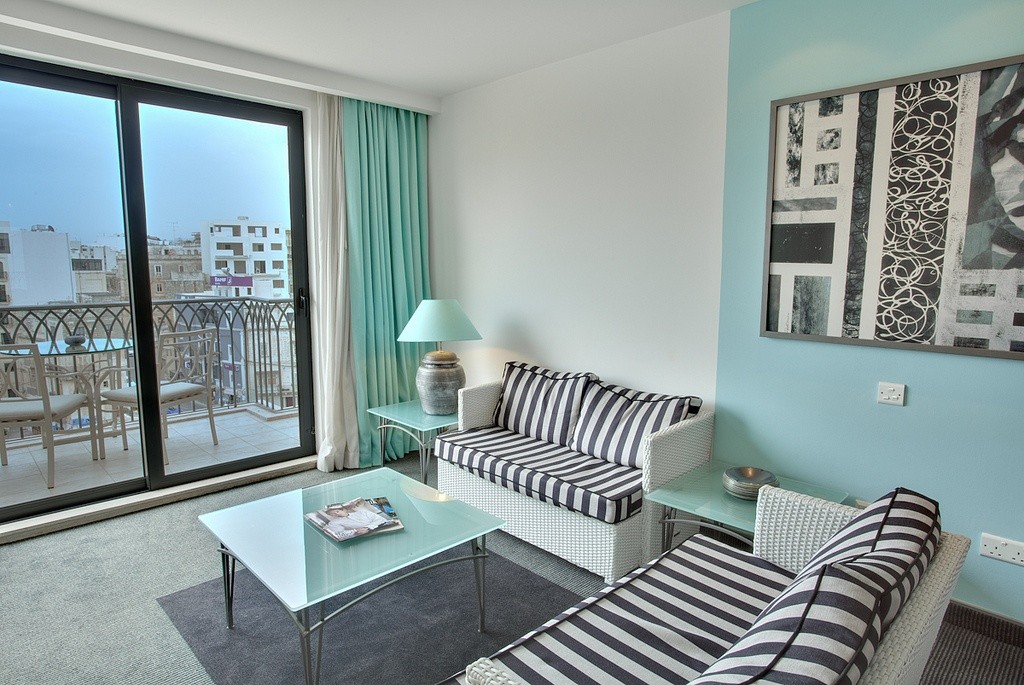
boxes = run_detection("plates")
[721,466,780,500]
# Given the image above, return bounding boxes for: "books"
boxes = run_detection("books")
[303,496,405,543]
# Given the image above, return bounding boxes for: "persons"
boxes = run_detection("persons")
[323,503,387,540]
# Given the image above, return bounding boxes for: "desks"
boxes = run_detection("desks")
[1,339,133,452]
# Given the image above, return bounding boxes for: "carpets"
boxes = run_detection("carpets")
[154,540,586,685]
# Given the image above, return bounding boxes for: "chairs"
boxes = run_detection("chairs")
[0,343,97,488]
[94,327,220,465]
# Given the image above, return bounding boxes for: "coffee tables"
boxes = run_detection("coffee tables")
[196,466,506,685]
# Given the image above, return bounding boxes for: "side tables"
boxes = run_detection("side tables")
[645,460,850,559]
[366,400,459,486]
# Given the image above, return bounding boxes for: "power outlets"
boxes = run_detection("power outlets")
[978,533,1024,567]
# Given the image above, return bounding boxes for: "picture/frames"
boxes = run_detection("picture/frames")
[759,55,1024,363]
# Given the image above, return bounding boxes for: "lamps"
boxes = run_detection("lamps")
[397,298,484,415]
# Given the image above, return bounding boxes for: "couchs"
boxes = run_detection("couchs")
[434,361,716,583]
[434,483,971,685]
